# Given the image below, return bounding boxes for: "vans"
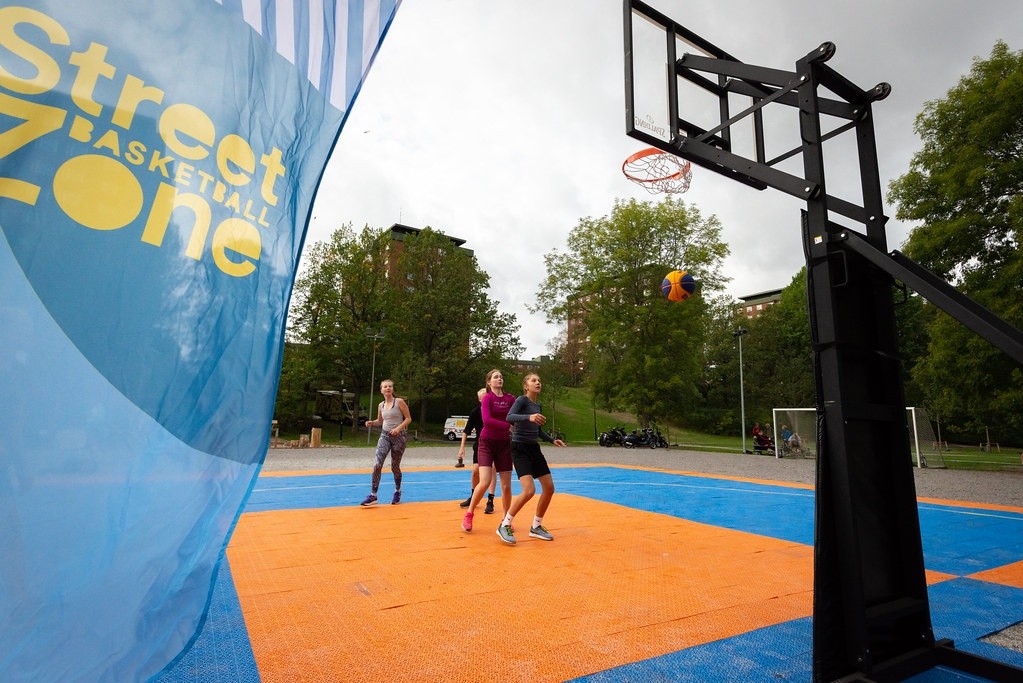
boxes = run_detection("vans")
[443,415,477,441]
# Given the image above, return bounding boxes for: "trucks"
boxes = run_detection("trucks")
[317,390,367,426]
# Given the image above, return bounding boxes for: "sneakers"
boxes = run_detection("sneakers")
[361,495,378,505]
[501,518,515,532]
[391,491,401,504]
[462,510,474,532]
[496,523,516,544]
[460,498,479,508]
[529,525,553,540]
[484,502,494,513]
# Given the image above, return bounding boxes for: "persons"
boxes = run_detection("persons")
[496,372,567,544]
[780,425,801,449]
[462,369,516,533]
[753,422,774,456]
[360,379,411,507]
[456,387,497,514]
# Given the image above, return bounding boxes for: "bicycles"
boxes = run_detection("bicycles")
[547,427,566,442]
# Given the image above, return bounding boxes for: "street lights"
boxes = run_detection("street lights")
[731,325,748,453]
[367,336,384,445]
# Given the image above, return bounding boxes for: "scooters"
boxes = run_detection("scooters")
[598,424,668,449]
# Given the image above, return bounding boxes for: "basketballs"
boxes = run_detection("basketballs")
[661,270,695,302]
